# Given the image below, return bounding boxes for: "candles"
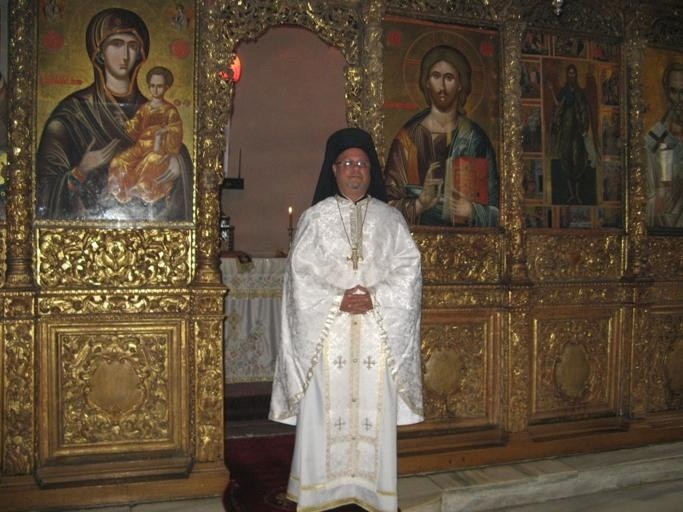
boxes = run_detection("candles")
[286,205,296,227]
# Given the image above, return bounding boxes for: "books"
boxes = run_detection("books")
[445,154,490,226]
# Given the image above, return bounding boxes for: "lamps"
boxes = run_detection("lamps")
[218,54,242,178]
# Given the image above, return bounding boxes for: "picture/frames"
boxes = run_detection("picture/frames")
[0,0,12,224]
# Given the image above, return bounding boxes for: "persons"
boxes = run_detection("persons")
[265,125,426,512]
[103,66,185,206]
[381,43,504,234]
[645,61,683,233]
[545,64,600,207]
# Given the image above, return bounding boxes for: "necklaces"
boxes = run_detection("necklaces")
[331,194,370,270]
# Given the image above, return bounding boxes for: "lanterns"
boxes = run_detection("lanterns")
[220,54,241,83]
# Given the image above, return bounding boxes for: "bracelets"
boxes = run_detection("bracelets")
[65,180,78,192]
[72,167,85,185]
[68,174,80,187]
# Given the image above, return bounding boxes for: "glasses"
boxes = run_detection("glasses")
[334,158,374,168]
